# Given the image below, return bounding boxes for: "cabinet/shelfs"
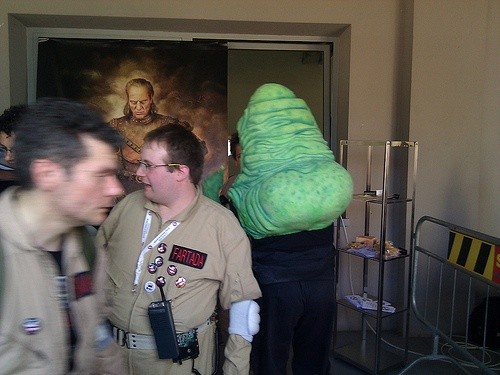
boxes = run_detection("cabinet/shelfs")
[332,139,418,373]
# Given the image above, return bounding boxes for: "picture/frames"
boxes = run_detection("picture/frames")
[25,26,341,195]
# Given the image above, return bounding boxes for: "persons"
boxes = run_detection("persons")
[0,104,36,193]
[219,129,260,222]
[227,83,353,375]
[0,98,122,375]
[91,124,261,375]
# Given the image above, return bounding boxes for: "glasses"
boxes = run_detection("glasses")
[0,147,11,152]
[139,159,180,169]
